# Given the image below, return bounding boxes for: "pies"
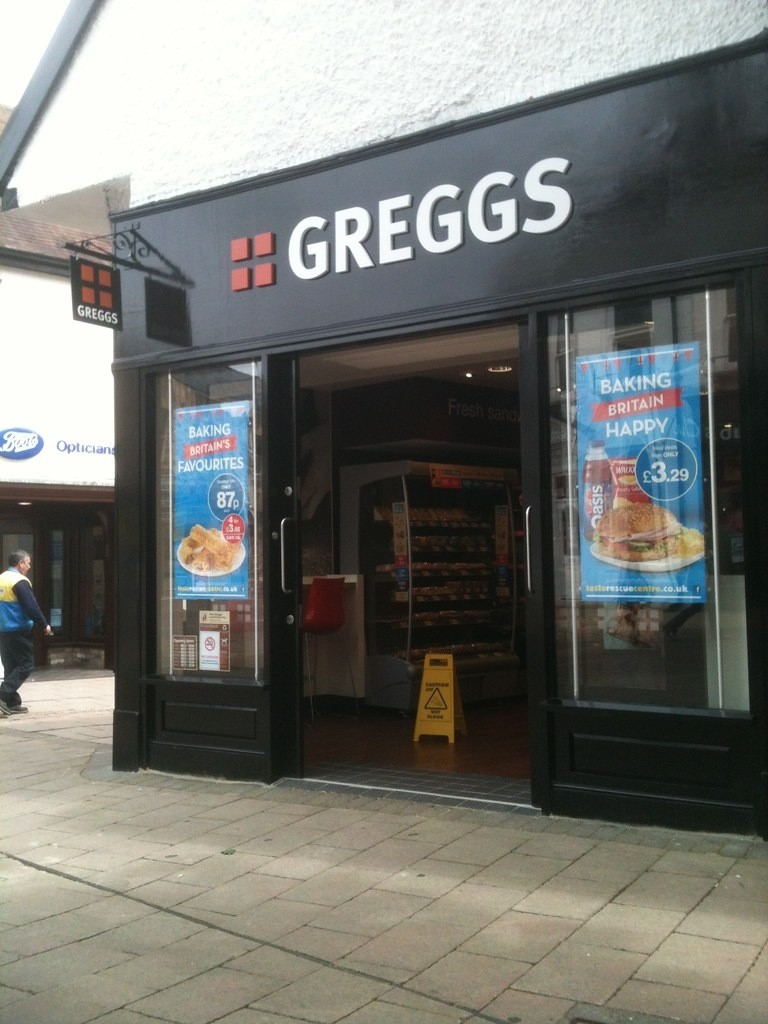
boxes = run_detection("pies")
[179,524,239,572]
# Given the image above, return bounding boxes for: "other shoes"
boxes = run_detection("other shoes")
[0,698,12,715]
[663,626,679,641]
[8,704,29,713]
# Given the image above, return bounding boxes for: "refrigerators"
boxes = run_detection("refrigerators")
[339,462,522,716]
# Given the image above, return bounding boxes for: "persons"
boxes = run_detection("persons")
[0,549,50,716]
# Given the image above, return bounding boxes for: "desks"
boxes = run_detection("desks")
[302,574,365,699]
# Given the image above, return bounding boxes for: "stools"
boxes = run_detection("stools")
[302,577,359,724]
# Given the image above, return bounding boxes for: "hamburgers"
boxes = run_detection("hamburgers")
[593,503,679,561]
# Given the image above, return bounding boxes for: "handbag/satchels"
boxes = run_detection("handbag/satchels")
[608,604,665,652]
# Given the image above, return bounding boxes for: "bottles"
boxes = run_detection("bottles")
[390,506,505,658]
[582,440,614,541]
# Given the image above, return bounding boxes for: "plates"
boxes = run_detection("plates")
[590,544,705,571]
[177,531,246,577]
